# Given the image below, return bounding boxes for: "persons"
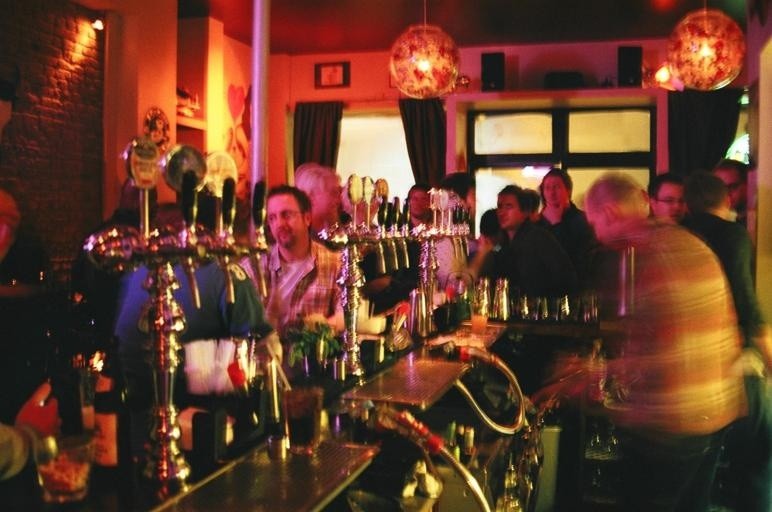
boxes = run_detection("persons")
[2,160,770,511]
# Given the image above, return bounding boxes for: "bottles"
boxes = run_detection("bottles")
[413,459,435,499]
[496,420,545,512]
[92,334,132,470]
[442,416,476,466]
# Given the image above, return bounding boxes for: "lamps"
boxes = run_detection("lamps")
[666,0,746,92]
[389,0,461,100]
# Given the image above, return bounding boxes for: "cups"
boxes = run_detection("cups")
[409,288,428,339]
[33,428,93,503]
[78,381,95,434]
[476,276,492,320]
[266,433,287,460]
[283,387,323,456]
[493,278,511,321]
[470,301,489,335]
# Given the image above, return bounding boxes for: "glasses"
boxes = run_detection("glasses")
[267,210,302,222]
[654,197,685,206]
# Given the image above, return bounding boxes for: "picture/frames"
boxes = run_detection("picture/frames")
[315,61,349,88]
